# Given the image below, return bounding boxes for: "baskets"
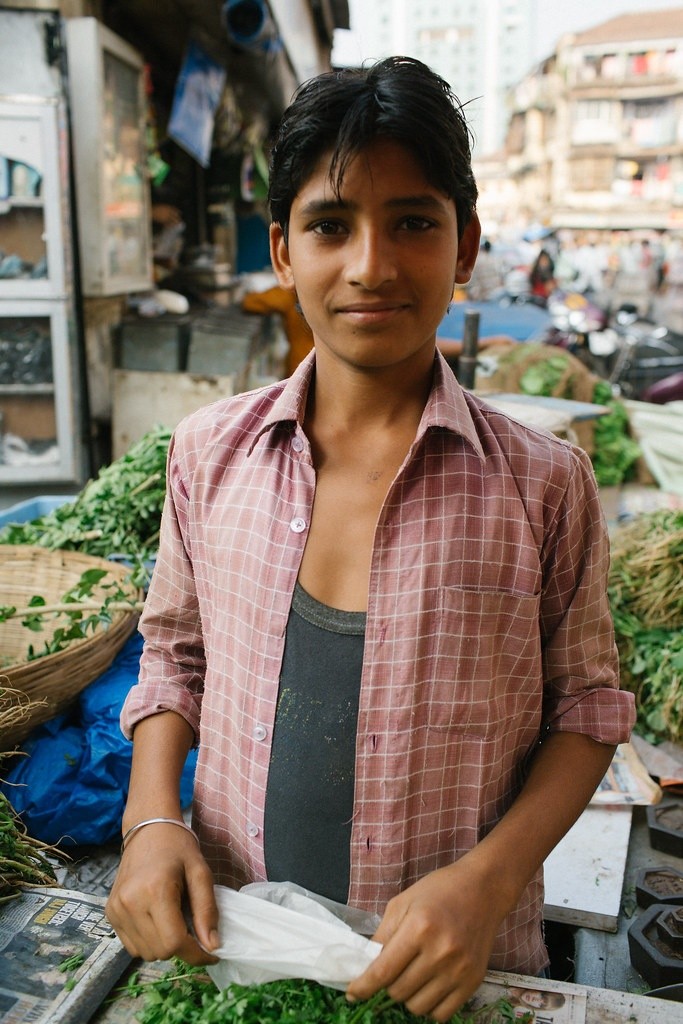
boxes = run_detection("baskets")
[1,544,144,731]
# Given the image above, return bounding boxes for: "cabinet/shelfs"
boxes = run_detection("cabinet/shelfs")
[1,101,85,484]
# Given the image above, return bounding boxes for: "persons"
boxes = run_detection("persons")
[436,335,517,359]
[467,228,683,309]
[105,54,638,1023]
[149,191,198,282]
[239,285,314,379]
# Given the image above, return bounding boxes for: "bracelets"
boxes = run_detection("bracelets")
[120,816,201,855]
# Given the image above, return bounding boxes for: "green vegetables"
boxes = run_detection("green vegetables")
[503,342,643,487]
[0,425,175,909]
[603,508,683,747]
[58,942,540,1024]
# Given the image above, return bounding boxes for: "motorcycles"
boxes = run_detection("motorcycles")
[543,259,683,405]
